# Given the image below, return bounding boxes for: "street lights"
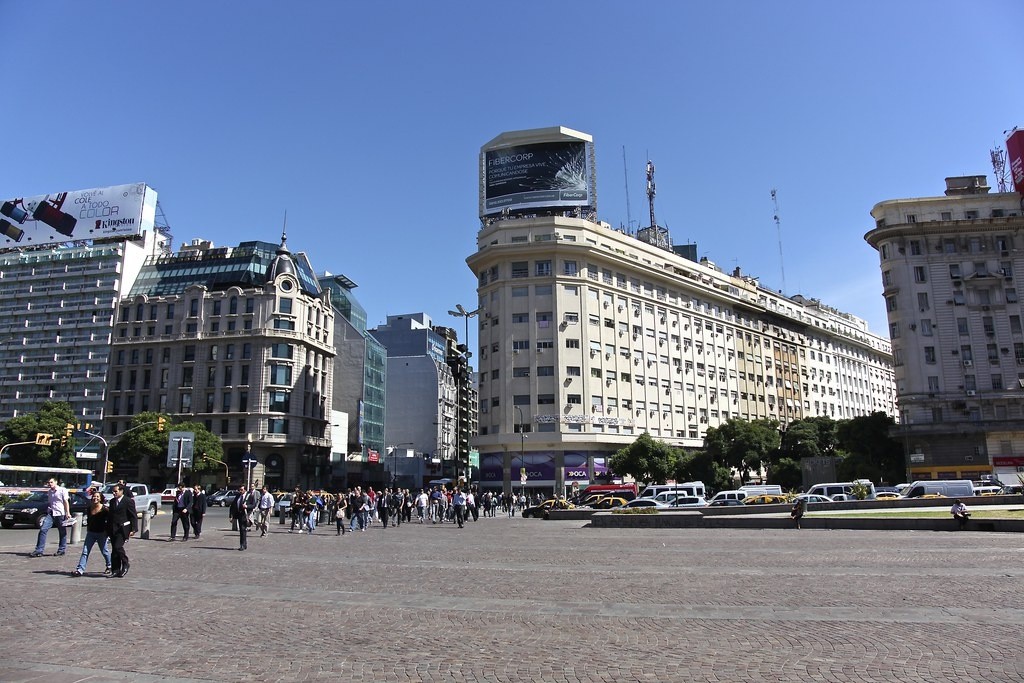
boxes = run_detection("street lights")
[515,405,523,509]
[313,425,339,490]
[903,397,916,485]
[432,304,485,516]
[395,442,445,489]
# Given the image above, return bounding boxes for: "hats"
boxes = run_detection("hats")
[377,491,381,493]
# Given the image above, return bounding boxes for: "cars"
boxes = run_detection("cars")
[522,495,834,518]
[0,491,91,529]
[206,490,334,517]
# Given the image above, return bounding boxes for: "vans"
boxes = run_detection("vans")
[161,488,193,503]
[568,485,637,504]
[707,478,1001,504]
[654,490,687,505]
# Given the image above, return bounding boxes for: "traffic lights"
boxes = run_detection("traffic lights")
[202,452,207,462]
[108,461,113,473]
[158,417,165,431]
[35,423,74,446]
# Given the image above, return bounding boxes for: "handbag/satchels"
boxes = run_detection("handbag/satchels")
[337,510,344,518]
[305,510,311,515]
[493,499,496,504]
[120,521,133,540]
[407,501,412,507]
[365,505,370,511]
[242,513,254,527]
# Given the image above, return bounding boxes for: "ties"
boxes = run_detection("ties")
[383,495,385,507]
[239,496,243,513]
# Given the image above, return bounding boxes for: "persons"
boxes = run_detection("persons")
[166,482,192,542]
[73,484,139,577]
[246,483,260,532]
[118,479,134,543]
[231,484,256,551]
[553,492,557,498]
[190,484,206,539]
[30,477,71,556]
[259,485,274,537]
[288,485,319,535]
[791,501,803,529]
[326,485,546,536]
[950,499,969,531]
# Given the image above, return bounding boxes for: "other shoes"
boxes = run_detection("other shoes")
[54,551,65,556]
[108,570,119,578]
[297,530,303,533]
[29,551,42,557]
[166,537,175,542]
[288,529,293,533]
[72,571,84,577]
[194,534,199,539]
[256,527,260,530]
[238,545,247,551]
[117,569,128,578]
[334,515,479,536]
[181,537,188,542]
[260,533,266,538]
[101,568,112,575]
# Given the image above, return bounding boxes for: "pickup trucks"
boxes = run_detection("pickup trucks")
[100,483,161,519]
[831,493,847,501]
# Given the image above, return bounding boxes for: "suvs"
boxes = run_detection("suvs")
[996,484,1024,496]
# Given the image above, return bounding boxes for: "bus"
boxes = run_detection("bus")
[639,480,705,500]
[428,478,457,491]
[0,465,92,507]
